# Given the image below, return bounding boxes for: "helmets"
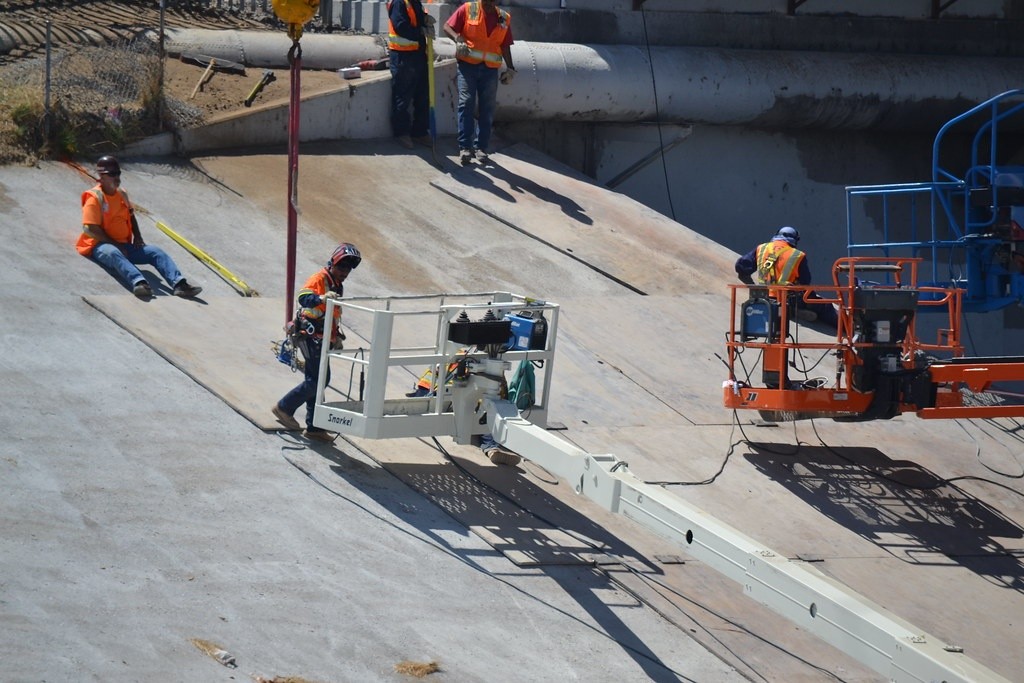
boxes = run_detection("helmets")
[330,242,361,269]
[95,157,119,173]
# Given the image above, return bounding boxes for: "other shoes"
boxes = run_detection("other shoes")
[795,310,818,321]
[459,148,473,162]
[473,144,488,162]
[488,448,522,467]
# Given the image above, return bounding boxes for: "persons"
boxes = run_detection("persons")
[734,226,825,390]
[386,0,438,150]
[270,241,361,440]
[76,156,203,299]
[443,0,515,163]
[402,346,521,467]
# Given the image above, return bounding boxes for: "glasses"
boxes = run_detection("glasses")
[107,171,122,177]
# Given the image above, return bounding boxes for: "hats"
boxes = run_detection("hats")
[774,227,798,246]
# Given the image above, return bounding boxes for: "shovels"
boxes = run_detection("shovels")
[244,69,274,105]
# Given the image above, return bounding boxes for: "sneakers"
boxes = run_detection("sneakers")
[414,134,434,147]
[175,283,202,296]
[274,407,298,429]
[304,431,334,441]
[393,135,414,150]
[135,281,153,296]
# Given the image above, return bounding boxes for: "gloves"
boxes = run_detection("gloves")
[501,67,518,85]
[454,37,470,59]
[421,26,436,41]
[321,290,340,304]
[424,15,436,27]
[334,336,345,356]
